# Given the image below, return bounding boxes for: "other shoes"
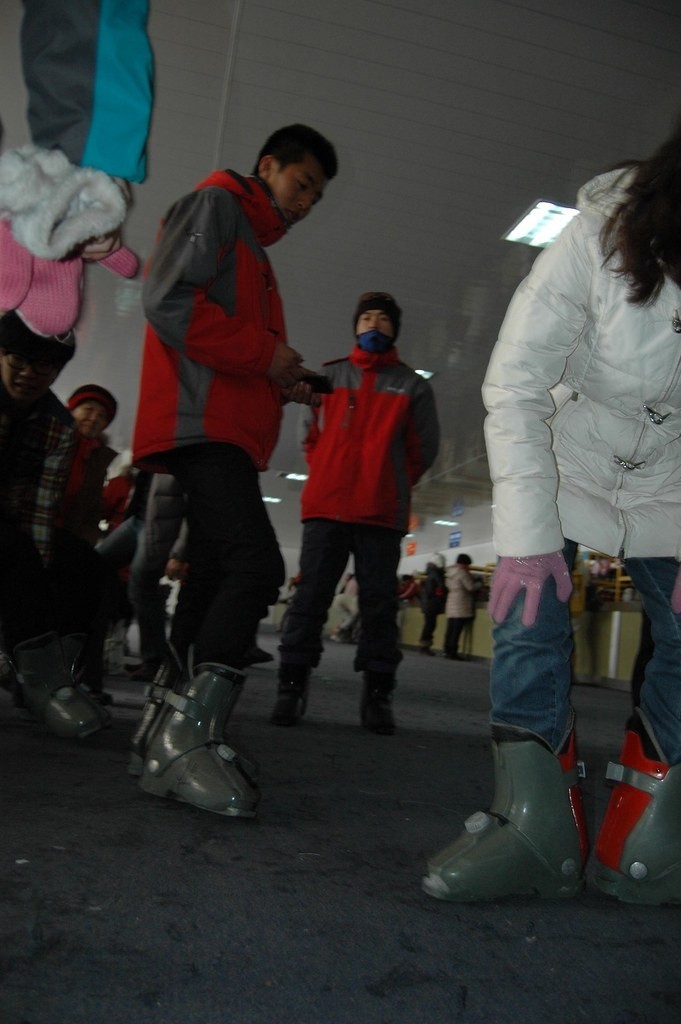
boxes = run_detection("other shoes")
[440,648,464,662]
[83,685,112,707]
[123,660,158,681]
[329,632,346,644]
[419,645,436,656]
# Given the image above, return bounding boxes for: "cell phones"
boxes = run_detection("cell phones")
[296,373,332,394]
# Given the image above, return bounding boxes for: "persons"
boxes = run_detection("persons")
[129,122,340,819]
[268,292,441,734]
[330,574,359,642]
[426,129,681,898]
[444,554,483,658]
[275,572,301,633]
[399,575,419,605]
[418,552,447,656]
[0,1,154,746]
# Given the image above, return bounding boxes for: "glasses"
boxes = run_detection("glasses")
[0,351,63,376]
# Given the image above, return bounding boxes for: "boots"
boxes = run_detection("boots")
[271,650,311,722]
[13,630,112,738]
[592,704,681,909]
[362,656,393,734]
[129,643,260,818]
[426,721,586,900]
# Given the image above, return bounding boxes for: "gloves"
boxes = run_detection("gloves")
[0,147,139,339]
[487,546,572,625]
[672,566,681,615]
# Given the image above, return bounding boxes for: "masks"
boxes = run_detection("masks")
[357,330,394,352]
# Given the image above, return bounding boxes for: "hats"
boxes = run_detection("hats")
[352,292,401,340]
[65,384,116,432]
